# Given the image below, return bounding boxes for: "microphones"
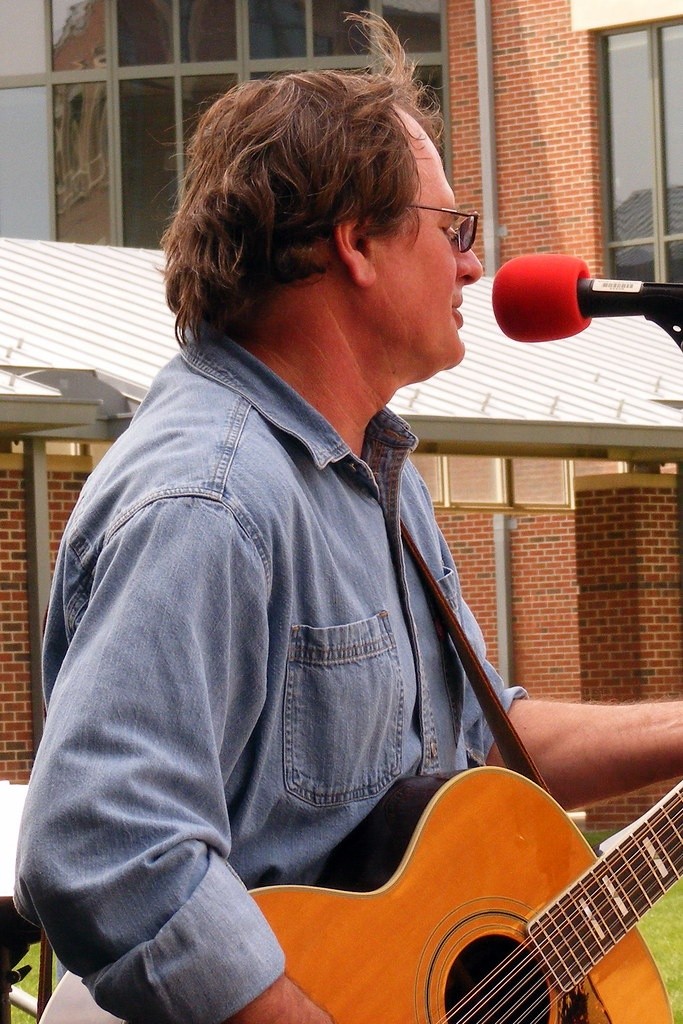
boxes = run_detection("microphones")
[492,253,683,343]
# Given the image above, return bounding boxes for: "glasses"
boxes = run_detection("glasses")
[405,199,479,252]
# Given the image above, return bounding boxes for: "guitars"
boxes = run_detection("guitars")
[34,764,683,1024]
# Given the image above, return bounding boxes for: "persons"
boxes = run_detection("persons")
[14,11,683,1024]
